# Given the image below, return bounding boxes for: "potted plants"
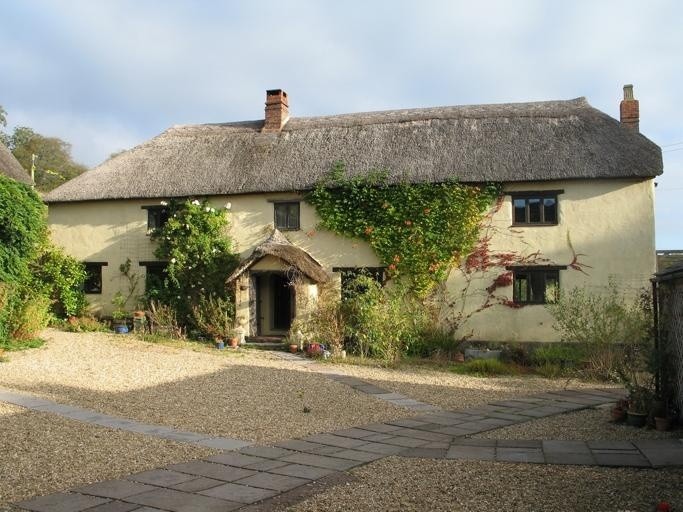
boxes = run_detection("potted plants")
[116,318,128,334]
[611,383,674,432]
[226,332,238,349]
[133,304,145,318]
[286,334,298,354]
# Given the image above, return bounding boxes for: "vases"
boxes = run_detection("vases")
[217,342,224,349]
